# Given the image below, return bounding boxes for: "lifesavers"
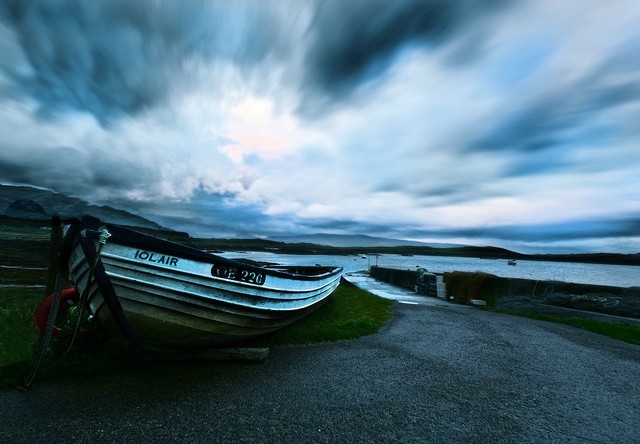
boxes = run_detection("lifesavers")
[34,288,86,338]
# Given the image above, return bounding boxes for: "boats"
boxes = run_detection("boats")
[22,214,343,387]
[508,260,516,265]
